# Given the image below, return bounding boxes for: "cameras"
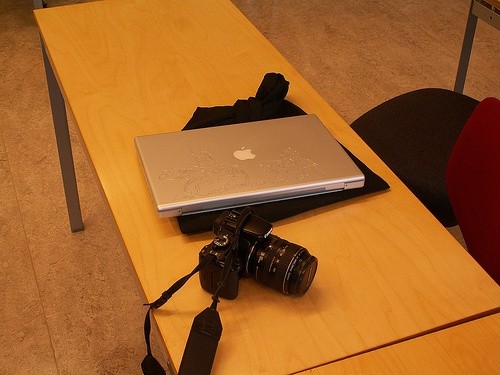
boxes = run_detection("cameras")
[200,206,318,300]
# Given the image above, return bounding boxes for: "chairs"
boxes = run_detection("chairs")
[350,1,499,228]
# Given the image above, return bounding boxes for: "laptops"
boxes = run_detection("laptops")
[135,114,365,219]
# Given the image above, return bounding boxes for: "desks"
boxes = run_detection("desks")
[32,1,500,375]
